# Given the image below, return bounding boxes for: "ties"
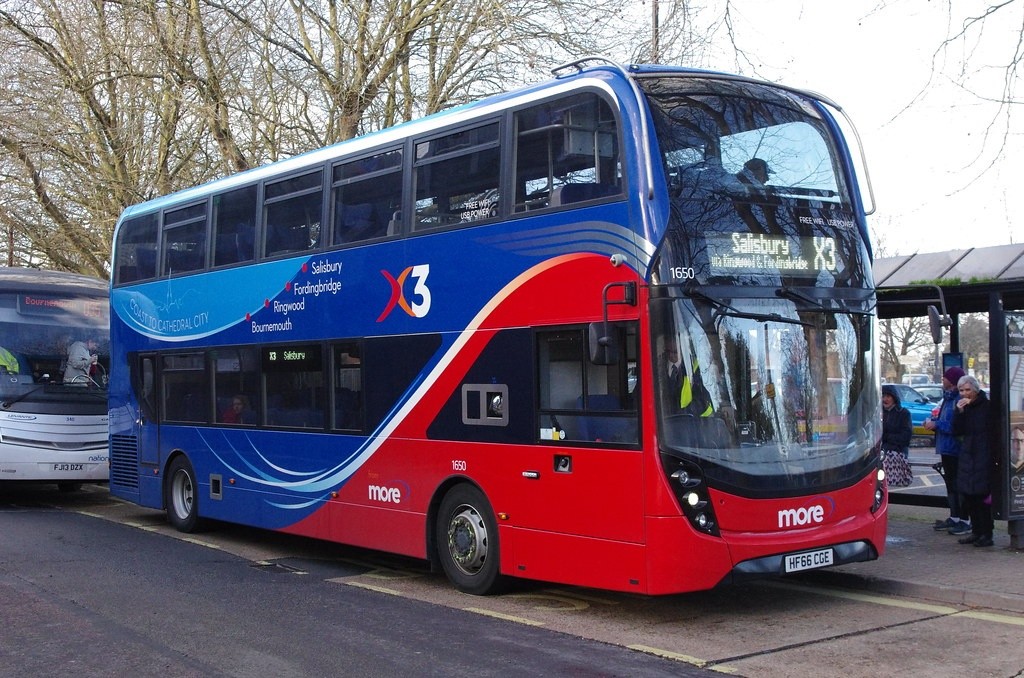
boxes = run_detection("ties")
[671,364,678,383]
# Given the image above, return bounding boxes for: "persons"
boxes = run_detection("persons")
[221,395,252,424]
[1011,426,1023,481]
[732,156,777,187]
[923,368,973,533]
[954,375,999,547]
[658,333,715,418]
[47,335,101,389]
[880,384,912,487]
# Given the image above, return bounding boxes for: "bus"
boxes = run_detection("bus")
[0,264,111,495]
[109,53,888,599]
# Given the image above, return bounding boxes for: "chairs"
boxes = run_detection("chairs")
[217,397,231,418]
[172,390,209,424]
[135,247,184,280]
[194,233,238,270]
[269,407,308,427]
[319,201,383,244]
[551,182,618,206]
[320,387,358,429]
[236,223,309,262]
[575,394,626,442]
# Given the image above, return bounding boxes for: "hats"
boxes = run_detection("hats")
[943,366,964,385]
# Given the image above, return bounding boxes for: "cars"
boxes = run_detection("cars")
[902,373,934,385]
[749,376,849,445]
[905,384,944,408]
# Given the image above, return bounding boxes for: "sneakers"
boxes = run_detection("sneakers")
[932,517,972,534]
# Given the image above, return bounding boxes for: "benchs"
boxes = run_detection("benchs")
[386,210,461,236]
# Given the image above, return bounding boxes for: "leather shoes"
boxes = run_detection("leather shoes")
[957,534,994,547]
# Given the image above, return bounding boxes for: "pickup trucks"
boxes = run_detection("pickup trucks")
[802,383,939,445]
